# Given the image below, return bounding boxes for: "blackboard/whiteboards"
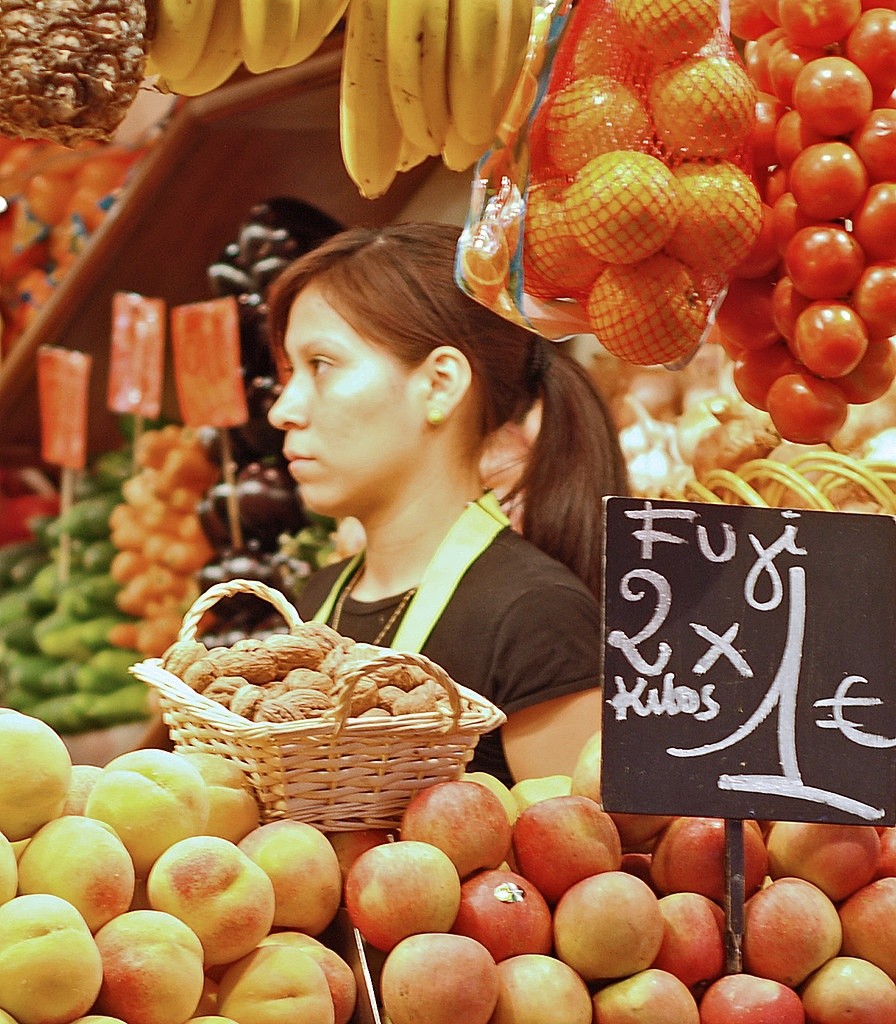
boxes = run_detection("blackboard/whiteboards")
[601,497,895,827]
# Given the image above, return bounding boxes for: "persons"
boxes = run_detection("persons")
[137,219,634,785]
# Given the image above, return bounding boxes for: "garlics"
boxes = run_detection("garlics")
[565,322,896,517]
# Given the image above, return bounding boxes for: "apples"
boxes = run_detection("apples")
[340,732,896,1024]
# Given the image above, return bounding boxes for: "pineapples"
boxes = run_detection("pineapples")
[1,1,158,148]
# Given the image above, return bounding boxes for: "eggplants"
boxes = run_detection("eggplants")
[193,202,312,626]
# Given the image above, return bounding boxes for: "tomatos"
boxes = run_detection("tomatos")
[710,0,896,446]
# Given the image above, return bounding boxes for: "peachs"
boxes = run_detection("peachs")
[0,707,358,1024]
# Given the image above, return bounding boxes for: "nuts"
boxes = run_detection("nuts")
[160,618,446,721]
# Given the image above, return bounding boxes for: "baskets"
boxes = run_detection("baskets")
[125,578,509,833]
[652,444,896,515]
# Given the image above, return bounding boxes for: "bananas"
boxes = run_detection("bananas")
[148,0,549,200]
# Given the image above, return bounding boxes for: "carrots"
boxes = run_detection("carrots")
[101,423,221,656]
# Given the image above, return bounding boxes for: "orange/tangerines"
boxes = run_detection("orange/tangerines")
[461,71,538,306]
[519,0,765,367]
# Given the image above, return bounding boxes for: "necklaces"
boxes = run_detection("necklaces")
[330,562,415,645]
